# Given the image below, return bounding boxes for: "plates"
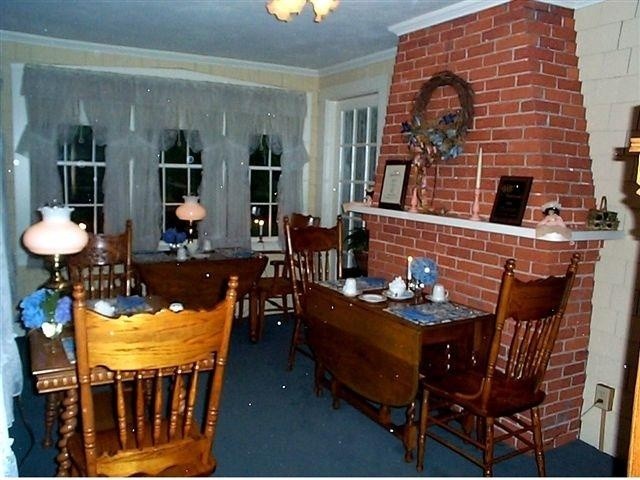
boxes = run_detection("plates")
[341,292,450,304]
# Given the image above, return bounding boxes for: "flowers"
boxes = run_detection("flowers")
[162,228,187,244]
[398,111,467,170]
[20,289,72,330]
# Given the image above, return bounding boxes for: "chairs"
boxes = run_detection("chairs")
[68,258,142,299]
[417,250,583,477]
[54,220,132,301]
[240,214,322,341]
[284,215,347,393]
[66,277,238,477]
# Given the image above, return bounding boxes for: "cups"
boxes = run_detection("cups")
[169,303,183,313]
[343,277,449,299]
[175,238,212,262]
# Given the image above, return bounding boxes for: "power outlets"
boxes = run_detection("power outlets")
[594,384,614,411]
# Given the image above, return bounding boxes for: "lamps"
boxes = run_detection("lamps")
[267,0,340,22]
[24,204,89,294]
[175,196,206,255]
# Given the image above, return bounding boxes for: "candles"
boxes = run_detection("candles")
[476,147,483,187]
